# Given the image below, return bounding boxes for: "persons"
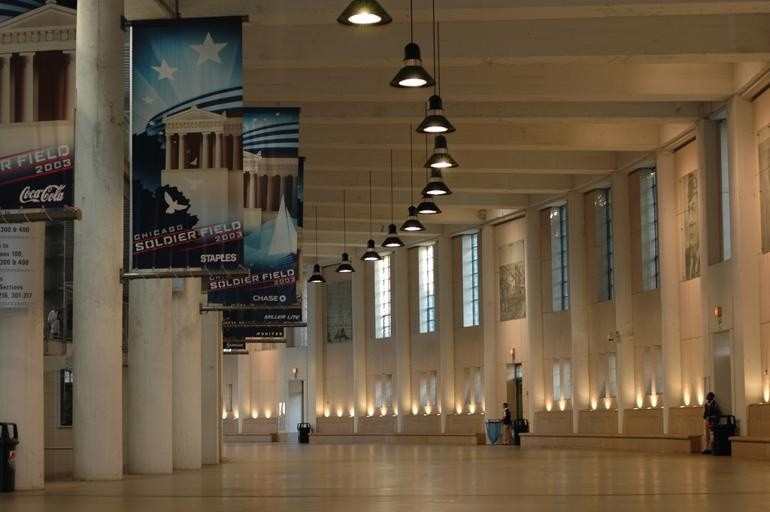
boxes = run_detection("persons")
[702,390,721,454]
[47,304,61,337]
[499,401,513,445]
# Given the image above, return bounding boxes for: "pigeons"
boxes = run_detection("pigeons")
[189,158,199,167]
[163,190,188,214]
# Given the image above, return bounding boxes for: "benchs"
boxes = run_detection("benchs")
[221,416,278,443]
[516,403,770,461]
[306,413,485,445]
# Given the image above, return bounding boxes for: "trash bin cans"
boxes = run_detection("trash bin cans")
[514,419,529,446]
[710,415,737,456]
[0,422,19,492]
[297,423,311,443]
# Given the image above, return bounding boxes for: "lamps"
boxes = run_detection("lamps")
[334,189,355,276]
[380,148,406,250]
[421,167,452,198]
[361,170,382,265]
[416,103,442,217]
[423,133,460,170]
[308,206,327,285]
[389,2,436,91]
[399,123,427,235]
[334,2,395,29]
[414,20,455,135]
